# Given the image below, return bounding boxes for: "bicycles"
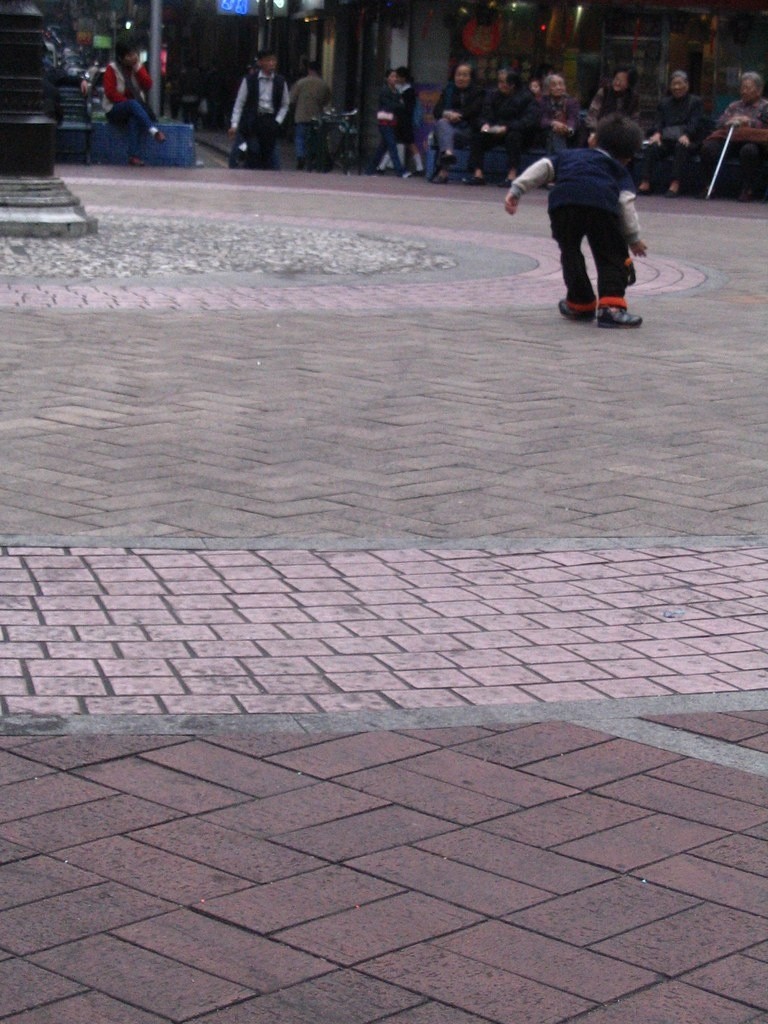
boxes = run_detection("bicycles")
[317,105,362,175]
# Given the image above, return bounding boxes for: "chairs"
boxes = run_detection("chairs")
[54,75,95,166]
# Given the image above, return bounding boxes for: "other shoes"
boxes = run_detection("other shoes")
[432,175,451,184]
[636,180,655,195]
[128,155,145,166]
[664,179,686,198]
[461,173,485,186]
[737,184,754,203]
[443,153,456,168]
[497,177,514,188]
[154,130,167,144]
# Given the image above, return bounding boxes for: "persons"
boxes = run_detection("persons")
[638,70,704,198]
[366,67,425,178]
[290,61,330,170]
[505,114,648,328]
[103,44,165,166]
[167,58,252,130]
[42,42,88,121]
[229,48,289,170]
[586,65,643,203]
[701,71,768,201]
[430,63,581,187]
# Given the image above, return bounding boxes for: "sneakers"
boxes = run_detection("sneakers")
[597,305,644,329]
[558,298,597,322]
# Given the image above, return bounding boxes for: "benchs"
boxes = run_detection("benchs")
[425,112,744,165]
[92,120,195,168]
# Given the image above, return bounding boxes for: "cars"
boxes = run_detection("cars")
[59,48,87,82]
